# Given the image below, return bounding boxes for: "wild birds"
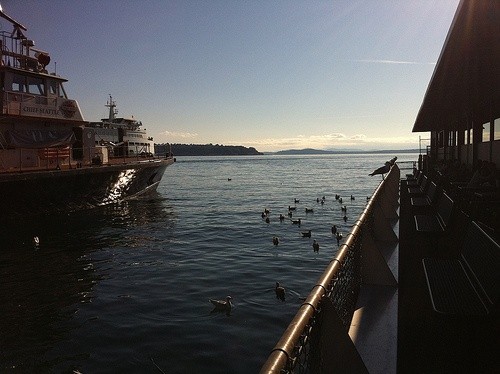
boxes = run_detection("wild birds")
[207,296,233,310]
[275,282,285,295]
[261,192,372,252]
[369,156,398,179]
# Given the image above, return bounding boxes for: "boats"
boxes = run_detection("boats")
[0,5,176,227]
